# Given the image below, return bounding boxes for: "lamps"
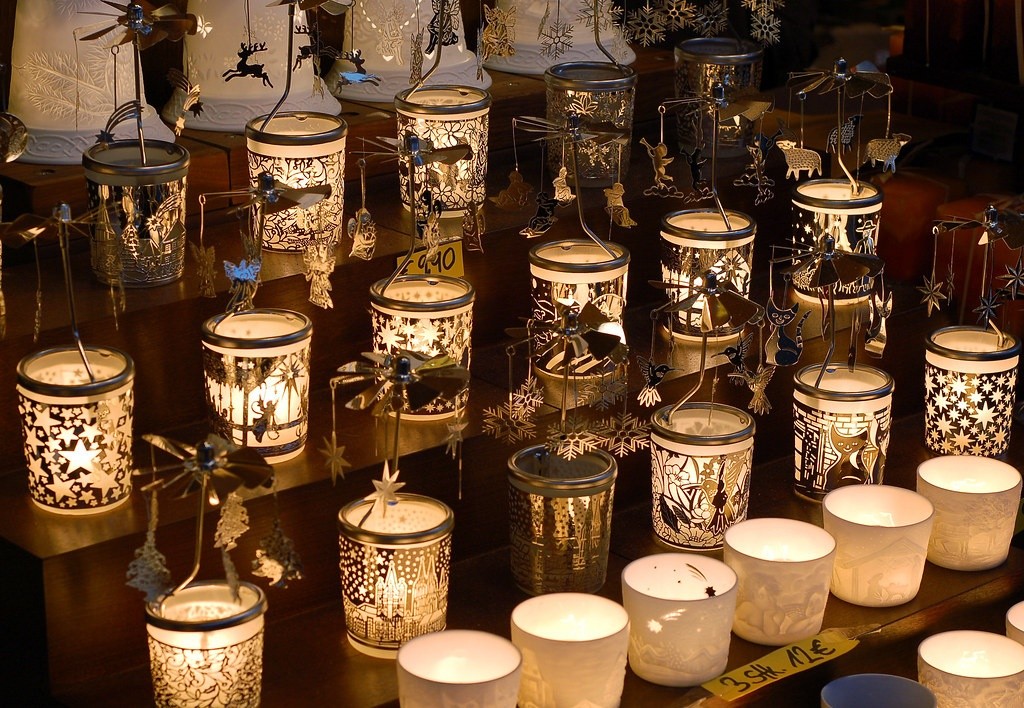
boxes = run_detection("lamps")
[0,0,1024,708]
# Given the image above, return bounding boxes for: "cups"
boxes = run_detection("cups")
[821,673,936,708]
